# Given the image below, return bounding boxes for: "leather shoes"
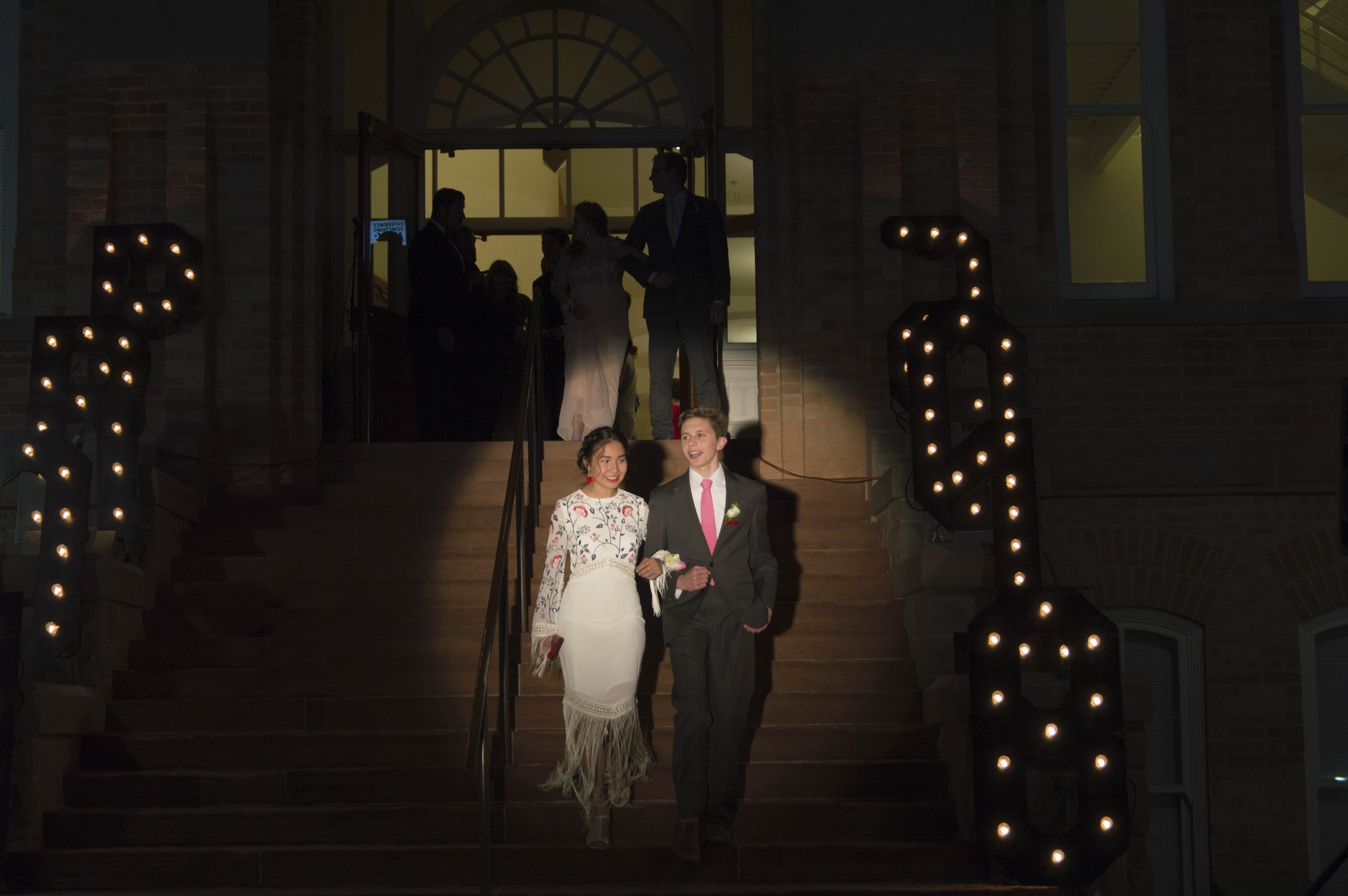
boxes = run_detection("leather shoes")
[703,824,732,846]
[671,821,703,863]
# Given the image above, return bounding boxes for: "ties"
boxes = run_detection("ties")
[699,479,718,586]
[665,198,677,248]
[446,233,466,269]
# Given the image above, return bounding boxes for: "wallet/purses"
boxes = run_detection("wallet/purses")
[547,634,564,659]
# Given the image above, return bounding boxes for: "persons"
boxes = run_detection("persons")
[620,149,734,433]
[379,186,689,442]
[641,405,781,863]
[530,427,685,853]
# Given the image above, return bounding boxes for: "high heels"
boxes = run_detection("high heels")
[585,814,613,850]
